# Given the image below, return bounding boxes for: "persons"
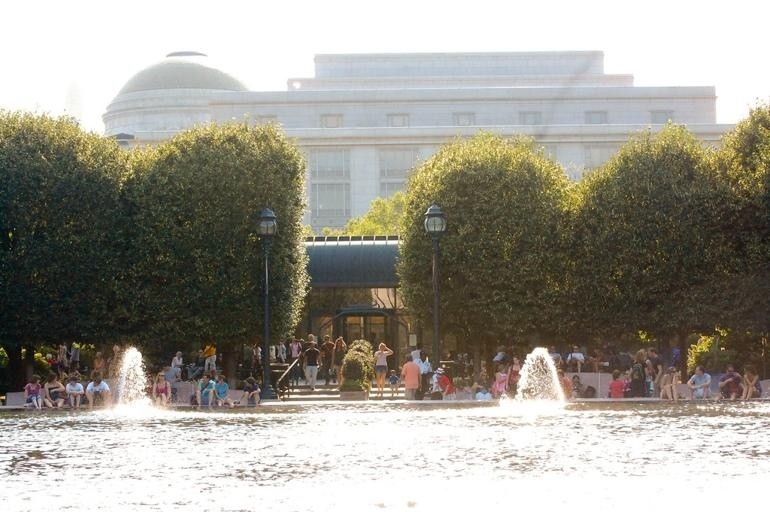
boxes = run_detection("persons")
[374,343,393,397]
[388,344,522,403]
[20,342,123,410]
[550,337,682,403]
[741,362,762,401]
[148,340,263,411]
[715,364,741,401]
[687,365,712,402]
[267,334,347,392]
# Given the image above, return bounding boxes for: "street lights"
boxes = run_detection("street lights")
[423,199,450,401]
[253,209,278,405]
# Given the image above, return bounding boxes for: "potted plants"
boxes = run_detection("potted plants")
[336,352,372,400]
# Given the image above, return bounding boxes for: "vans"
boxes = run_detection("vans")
[438,359,456,378]
